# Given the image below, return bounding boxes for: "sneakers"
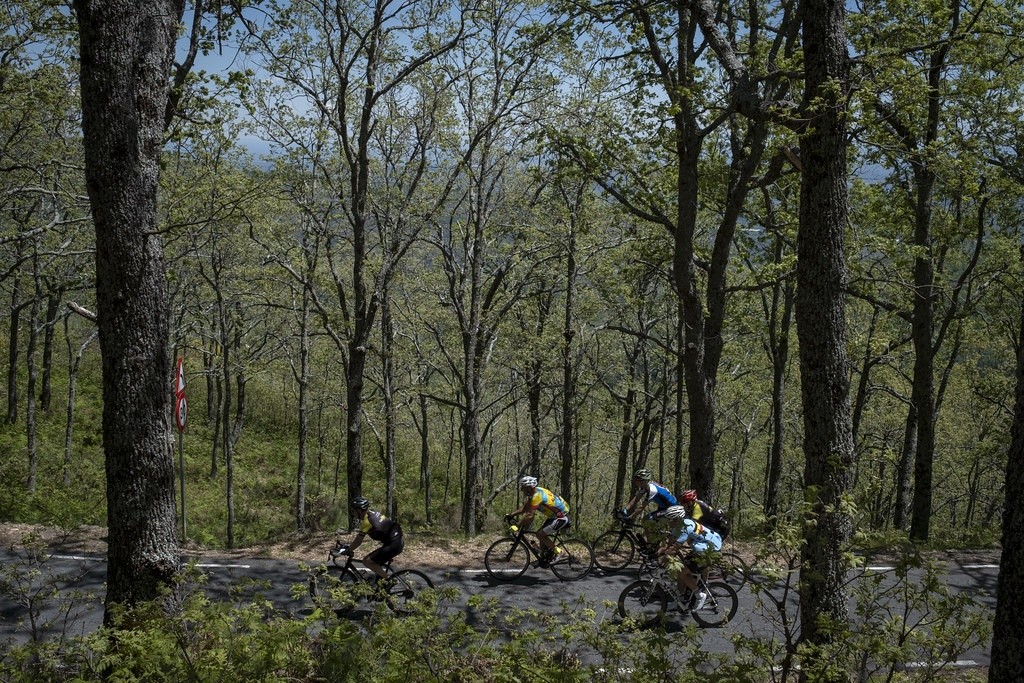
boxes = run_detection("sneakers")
[550,550,567,565]
[692,593,707,613]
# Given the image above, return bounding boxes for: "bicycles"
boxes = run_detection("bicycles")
[617,542,739,629]
[637,528,749,597]
[484,514,595,582]
[592,503,663,571]
[310,539,435,616]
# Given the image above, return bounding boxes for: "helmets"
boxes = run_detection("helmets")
[351,497,370,514]
[667,506,685,520]
[632,470,651,483]
[519,476,536,490]
[680,489,697,502]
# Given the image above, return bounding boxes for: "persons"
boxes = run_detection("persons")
[335,497,404,587]
[505,476,572,565]
[623,468,678,557]
[657,505,722,614]
[679,490,731,561]
